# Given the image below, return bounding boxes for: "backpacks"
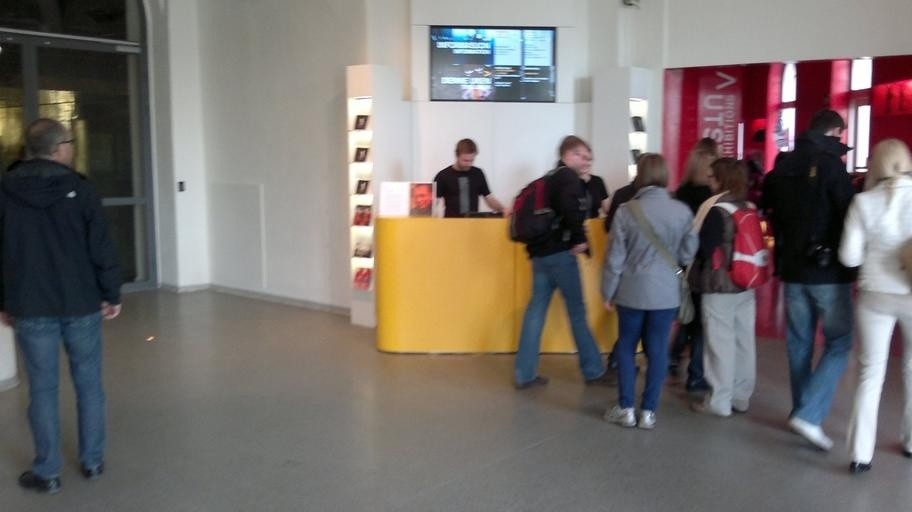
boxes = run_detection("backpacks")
[711,200,771,293]
[509,167,567,243]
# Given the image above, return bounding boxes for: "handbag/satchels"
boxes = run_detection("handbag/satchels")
[677,273,694,326]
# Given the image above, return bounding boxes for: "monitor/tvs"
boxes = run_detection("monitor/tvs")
[430,25,556,103]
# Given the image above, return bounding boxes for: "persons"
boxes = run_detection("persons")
[1,118,128,497]
[837,137,911,478]
[461,83,492,100]
[760,108,857,451]
[409,183,433,216]
[433,139,514,218]
[510,127,777,431]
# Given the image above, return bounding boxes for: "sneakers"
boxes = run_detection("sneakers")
[637,410,656,430]
[585,373,618,388]
[686,379,708,392]
[514,375,547,389]
[691,401,706,412]
[788,416,834,452]
[605,404,637,428]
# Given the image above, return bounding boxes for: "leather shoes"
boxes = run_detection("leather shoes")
[19,471,62,495]
[81,462,104,480]
